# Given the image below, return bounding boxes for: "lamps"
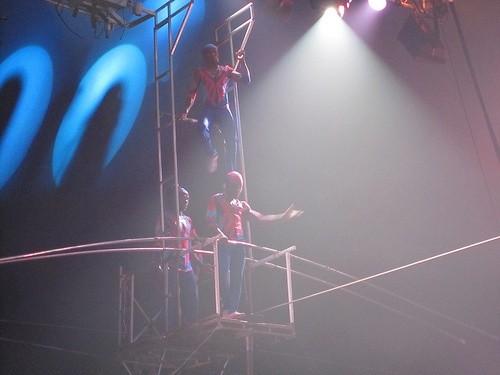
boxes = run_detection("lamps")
[47,0,453,42]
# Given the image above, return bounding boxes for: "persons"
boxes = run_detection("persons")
[207,170,304,322]
[182,43,252,173]
[155,185,215,323]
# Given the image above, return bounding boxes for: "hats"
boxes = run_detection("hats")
[224,171,243,184]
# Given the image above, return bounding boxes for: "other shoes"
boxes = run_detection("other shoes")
[207,149,219,172]
[220,311,244,320]
